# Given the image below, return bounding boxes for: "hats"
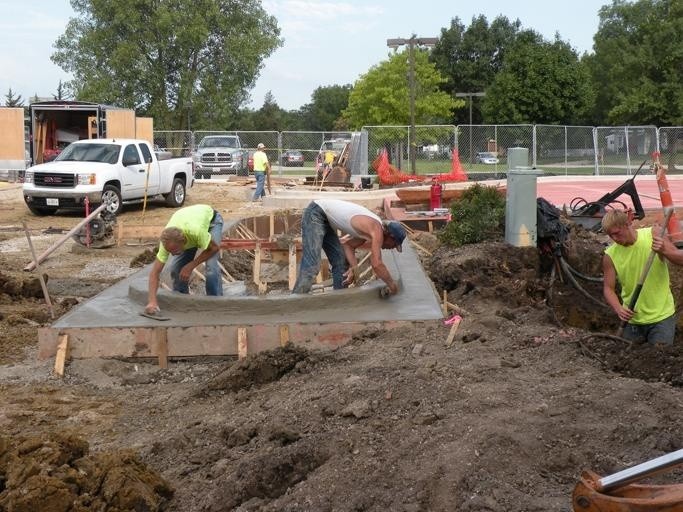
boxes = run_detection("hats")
[387,221,406,253]
[258,143,266,149]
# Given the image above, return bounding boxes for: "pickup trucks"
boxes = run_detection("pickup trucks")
[22,139,195,216]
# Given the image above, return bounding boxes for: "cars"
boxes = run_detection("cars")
[474,152,499,165]
[282,150,304,166]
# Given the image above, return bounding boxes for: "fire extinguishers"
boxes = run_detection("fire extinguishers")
[430,177,442,211]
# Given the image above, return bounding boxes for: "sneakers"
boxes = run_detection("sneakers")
[253,199,263,202]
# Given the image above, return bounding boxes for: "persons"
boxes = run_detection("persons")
[143,204,223,316]
[251,143,270,202]
[291,198,406,297]
[600,208,682,347]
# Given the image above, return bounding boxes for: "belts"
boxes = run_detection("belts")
[211,209,217,223]
[310,201,328,219]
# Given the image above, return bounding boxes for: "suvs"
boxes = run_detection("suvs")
[191,134,249,178]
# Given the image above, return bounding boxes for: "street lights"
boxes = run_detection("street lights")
[386,35,438,176]
[453,92,489,163]
